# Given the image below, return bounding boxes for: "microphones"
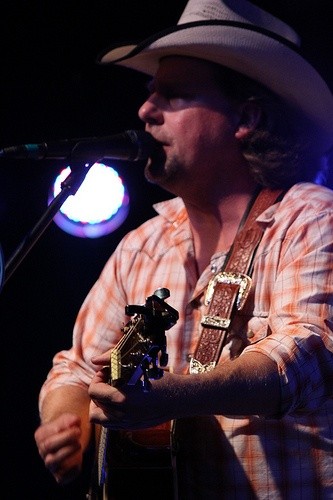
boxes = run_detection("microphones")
[0,128,155,163]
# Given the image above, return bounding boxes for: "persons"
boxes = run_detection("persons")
[33,1,331,499]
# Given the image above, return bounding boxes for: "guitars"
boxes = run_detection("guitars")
[87,288,180,500]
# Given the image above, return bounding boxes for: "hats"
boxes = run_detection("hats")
[99,0,333,161]
